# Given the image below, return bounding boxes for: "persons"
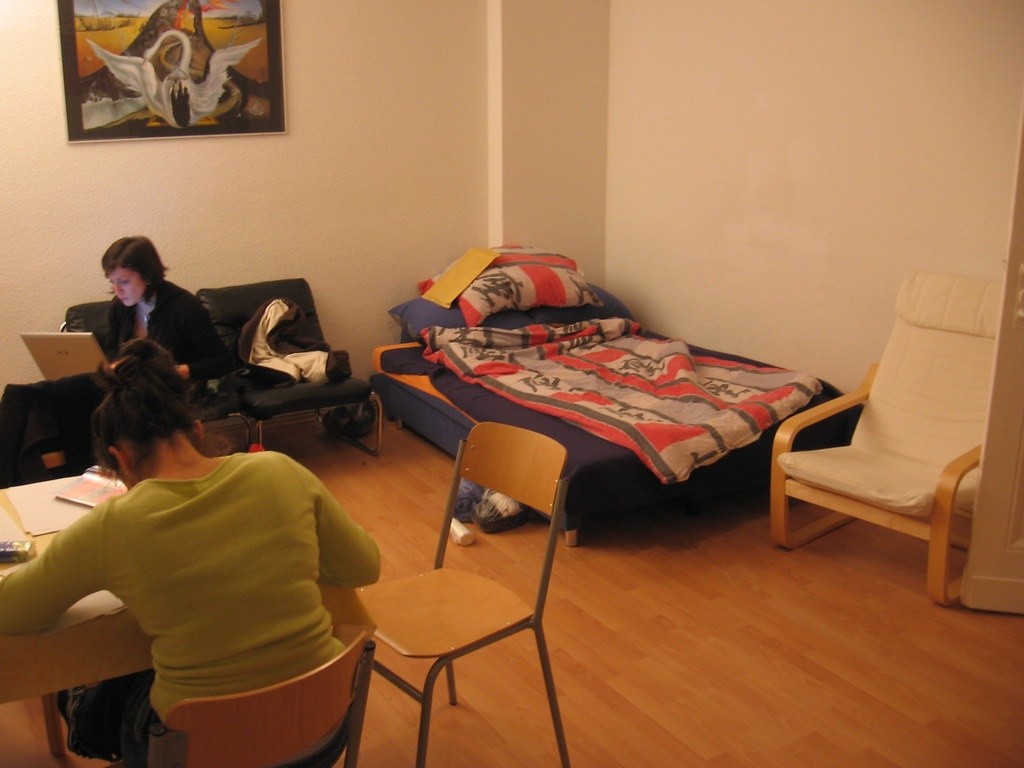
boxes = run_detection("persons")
[0,339,380,768]
[59,237,231,471]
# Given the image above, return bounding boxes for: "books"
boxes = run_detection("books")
[54,465,129,508]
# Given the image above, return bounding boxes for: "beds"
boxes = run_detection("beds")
[371,328,864,548]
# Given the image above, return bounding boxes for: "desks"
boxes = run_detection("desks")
[0,488,355,768]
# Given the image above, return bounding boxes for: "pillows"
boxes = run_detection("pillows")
[436,245,605,329]
[388,297,538,344]
[526,283,634,327]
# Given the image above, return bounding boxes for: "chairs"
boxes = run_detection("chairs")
[198,277,384,457]
[770,268,1007,607]
[61,299,254,452]
[147,629,376,768]
[356,421,570,768]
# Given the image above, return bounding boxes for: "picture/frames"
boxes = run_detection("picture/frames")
[57,0,289,144]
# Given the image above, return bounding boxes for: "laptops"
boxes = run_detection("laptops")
[20,332,111,381]
